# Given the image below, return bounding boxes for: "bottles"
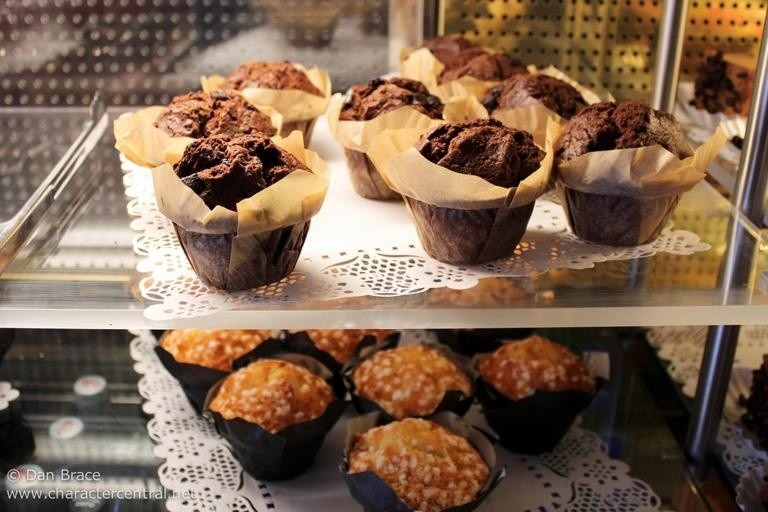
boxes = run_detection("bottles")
[0,380,37,480]
[46,416,105,512]
[72,374,131,501]
[5,464,46,512]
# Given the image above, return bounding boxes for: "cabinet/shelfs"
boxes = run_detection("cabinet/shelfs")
[0,0,768,512]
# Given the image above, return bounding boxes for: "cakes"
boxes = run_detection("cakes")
[446,53,532,84]
[156,330,274,417]
[404,38,487,82]
[154,134,329,289]
[153,93,275,138]
[345,418,501,511]
[297,330,394,365]
[327,79,448,198]
[474,336,603,456]
[368,119,552,264]
[202,352,355,480]
[553,100,726,245]
[343,345,476,420]
[200,62,331,150]
[474,74,588,126]
